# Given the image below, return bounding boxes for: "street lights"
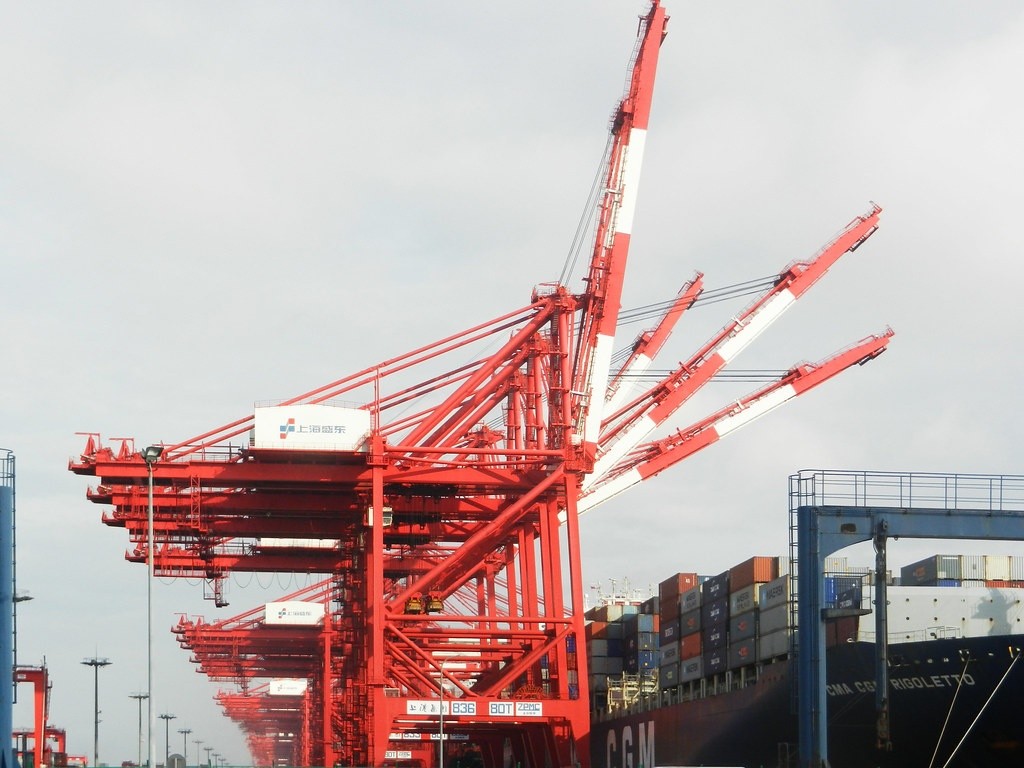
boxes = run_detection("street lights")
[195,740,229,768]
[130,694,148,768]
[179,730,191,767]
[81,657,114,768]
[159,714,176,768]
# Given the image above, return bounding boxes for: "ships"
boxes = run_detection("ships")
[439,557,1024,768]
